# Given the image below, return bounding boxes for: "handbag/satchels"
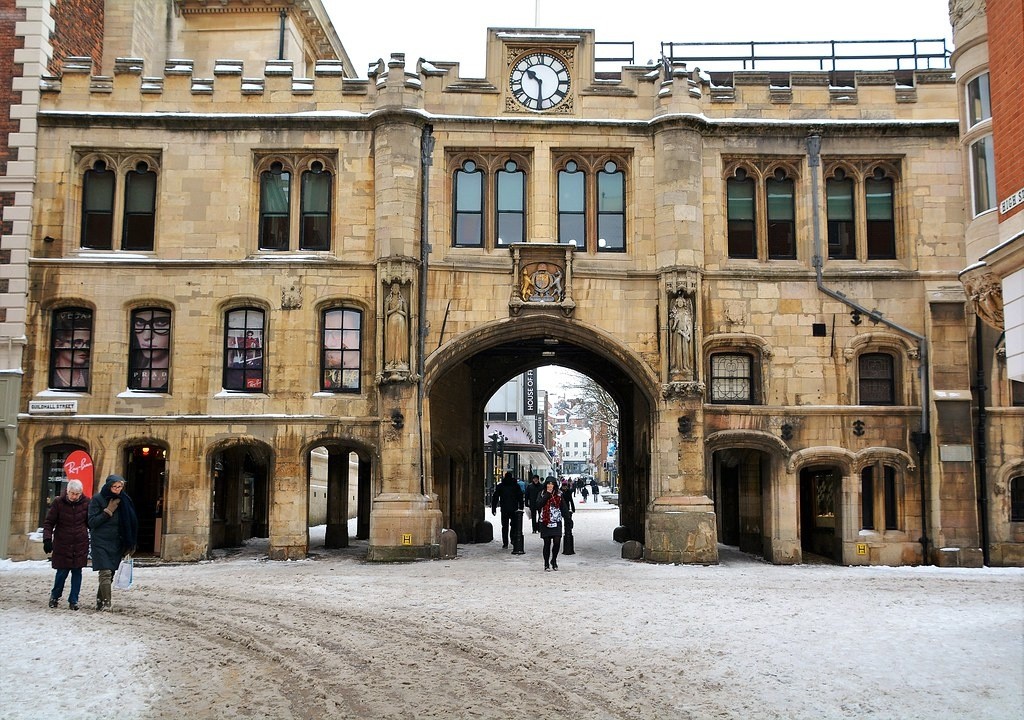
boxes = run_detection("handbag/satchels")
[114,555,133,589]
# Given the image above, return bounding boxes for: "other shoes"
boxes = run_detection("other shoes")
[49,595,58,609]
[70,602,79,611]
[545,563,550,571]
[551,560,558,571]
[502,545,508,549]
[95,603,111,612]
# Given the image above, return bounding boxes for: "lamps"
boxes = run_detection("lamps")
[526,431,531,436]
[521,427,527,433]
[529,435,533,439]
[530,438,534,442]
[486,422,490,429]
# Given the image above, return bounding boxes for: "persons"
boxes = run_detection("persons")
[492,470,599,549]
[135,311,170,388]
[43,479,90,610]
[88,475,137,611]
[536,476,570,572]
[53,319,90,387]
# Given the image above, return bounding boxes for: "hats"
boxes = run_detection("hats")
[532,475,539,480]
[106,475,123,487]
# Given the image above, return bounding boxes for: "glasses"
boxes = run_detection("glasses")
[69,491,80,496]
[110,485,123,490]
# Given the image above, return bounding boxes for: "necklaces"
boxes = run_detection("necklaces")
[57,367,82,386]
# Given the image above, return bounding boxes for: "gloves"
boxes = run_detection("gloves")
[104,498,120,517]
[43,538,54,554]
[572,507,575,513]
[125,549,136,556]
[543,492,552,501]
[525,503,528,507]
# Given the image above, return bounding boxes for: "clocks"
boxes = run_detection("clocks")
[506,48,573,112]
